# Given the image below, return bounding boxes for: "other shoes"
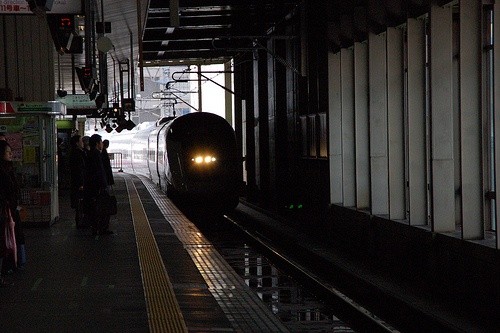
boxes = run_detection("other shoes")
[92,231,106,235]
[103,230,114,234]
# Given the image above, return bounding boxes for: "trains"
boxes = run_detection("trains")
[85,110,244,210]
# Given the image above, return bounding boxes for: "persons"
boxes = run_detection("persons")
[0,136,24,274]
[70,131,117,237]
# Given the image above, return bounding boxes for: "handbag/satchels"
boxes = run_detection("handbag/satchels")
[71,191,96,209]
[90,195,117,215]
